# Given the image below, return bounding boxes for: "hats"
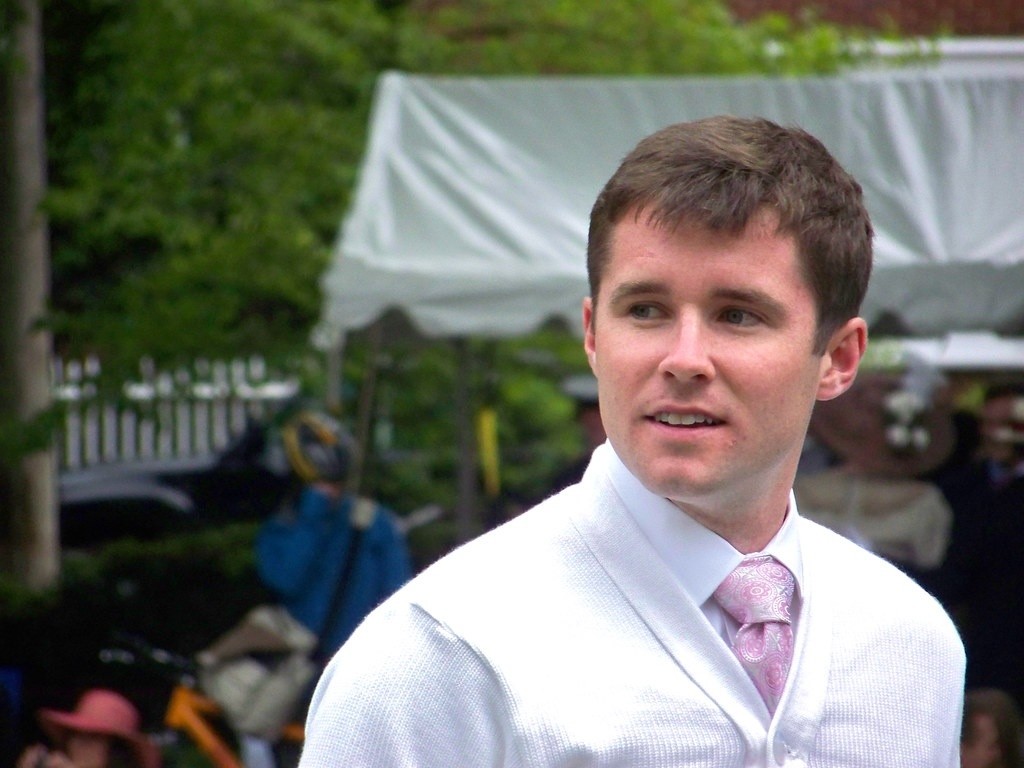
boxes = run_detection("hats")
[41,691,159,768]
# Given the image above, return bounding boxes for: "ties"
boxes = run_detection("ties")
[713,554,797,718]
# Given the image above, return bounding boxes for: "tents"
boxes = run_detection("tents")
[318,66,1023,472]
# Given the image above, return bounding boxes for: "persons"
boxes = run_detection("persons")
[295,115,968,768]
[794,382,1024,768]
